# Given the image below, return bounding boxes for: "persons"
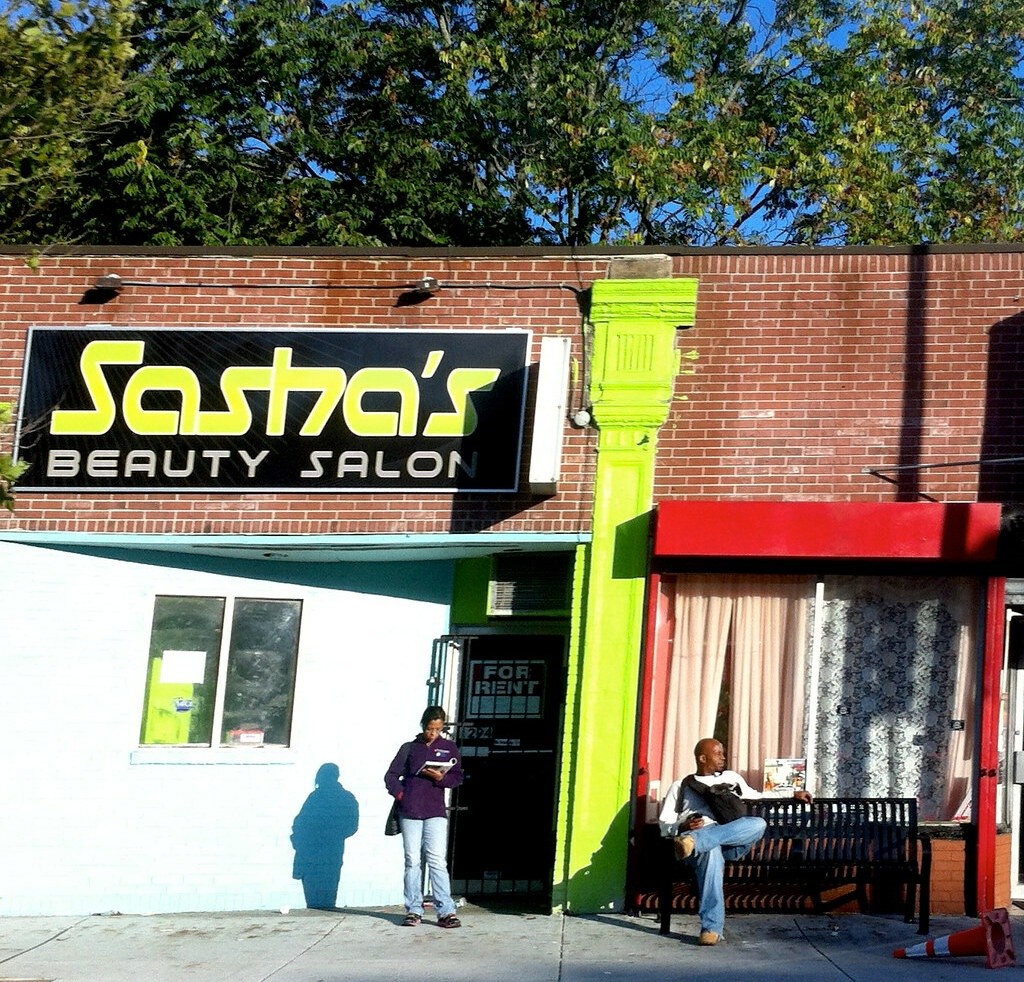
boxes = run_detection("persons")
[658,739,813,945]
[384,706,462,928]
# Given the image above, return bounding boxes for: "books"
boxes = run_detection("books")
[415,757,458,777]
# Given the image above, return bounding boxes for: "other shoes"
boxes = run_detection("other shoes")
[673,835,695,861]
[401,913,422,926]
[437,913,461,928]
[700,926,720,945]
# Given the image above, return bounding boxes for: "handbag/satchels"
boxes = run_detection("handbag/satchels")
[681,775,748,825]
[385,800,401,836]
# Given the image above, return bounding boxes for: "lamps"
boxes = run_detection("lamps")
[95,277,122,291]
[416,279,440,294]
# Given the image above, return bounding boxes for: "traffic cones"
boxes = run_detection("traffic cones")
[891,906,1018,968]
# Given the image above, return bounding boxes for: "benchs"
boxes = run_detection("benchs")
[657,798,932,936]
